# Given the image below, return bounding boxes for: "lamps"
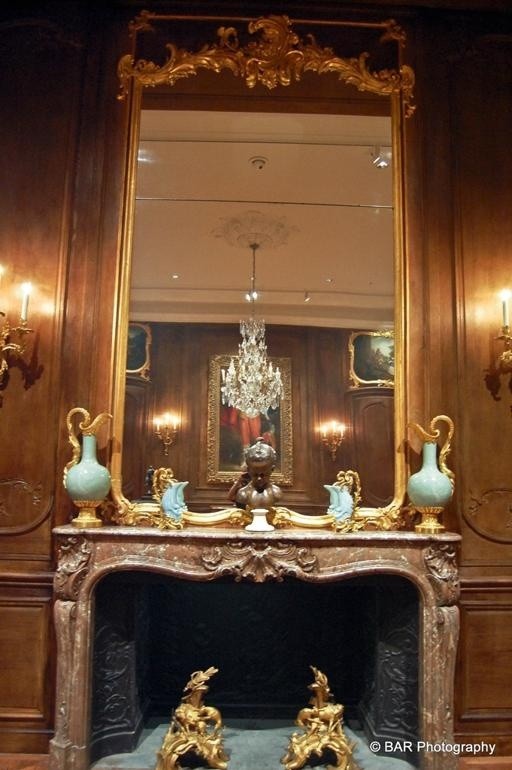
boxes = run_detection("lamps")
[209,207,300,422]
[2,263,35,377]
[488,286,512,399]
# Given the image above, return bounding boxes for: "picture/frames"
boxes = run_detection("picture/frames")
[346,327,396,392]
[124,319,154,387]
[206,349,296,489]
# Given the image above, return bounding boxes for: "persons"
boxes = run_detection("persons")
[236,437,284,508]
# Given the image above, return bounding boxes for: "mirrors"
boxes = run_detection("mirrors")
[58,1,426,527]
